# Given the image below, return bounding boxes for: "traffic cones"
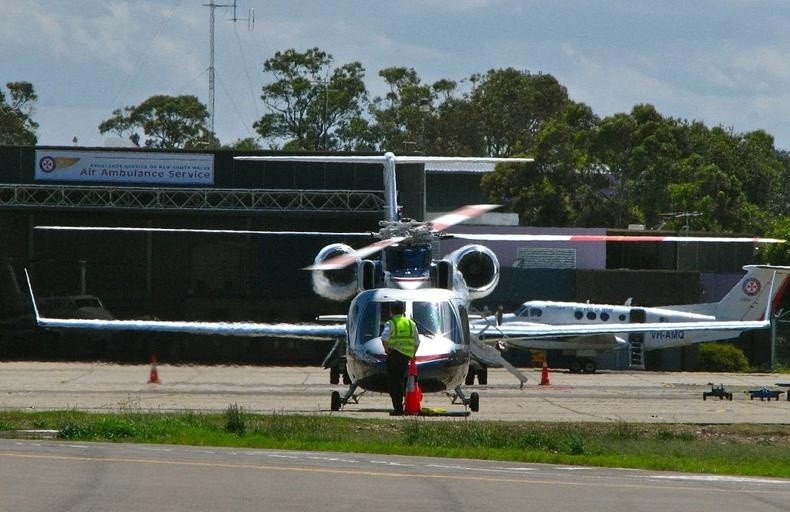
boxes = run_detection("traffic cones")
[146,353,161,385]
[403,356,424,417]
[538,358,551,387]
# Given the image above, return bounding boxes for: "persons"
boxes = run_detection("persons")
[381,300,421,416]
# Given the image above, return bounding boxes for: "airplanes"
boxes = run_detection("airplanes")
[25,154,786,414]
[469,264,789,374]
[1,262,164,343]
